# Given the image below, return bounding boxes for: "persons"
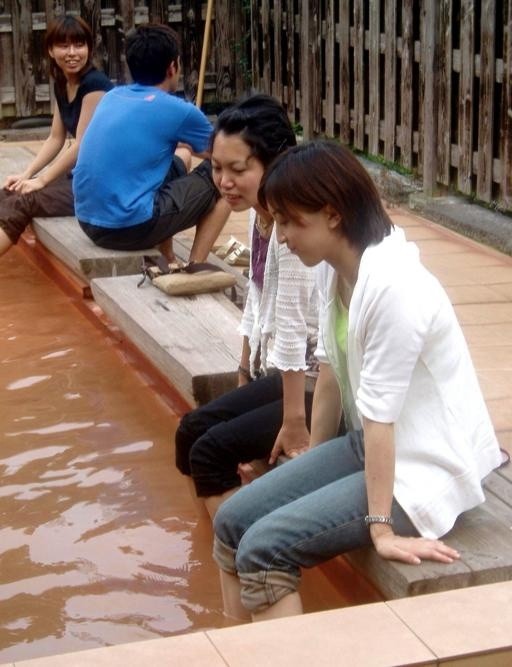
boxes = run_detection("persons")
[0,14,115,258]
[173,94,339,525]
[212,142,502,627]
[72,24,233,273]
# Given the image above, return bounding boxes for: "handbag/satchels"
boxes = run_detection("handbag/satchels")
[137,255,237,303]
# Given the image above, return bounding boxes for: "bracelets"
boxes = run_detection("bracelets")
[238,366,260,383]
[364,514,393,527]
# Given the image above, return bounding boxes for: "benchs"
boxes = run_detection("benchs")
[256,444,512,598]
[31,212,172,298]
[89,268,324,407]
